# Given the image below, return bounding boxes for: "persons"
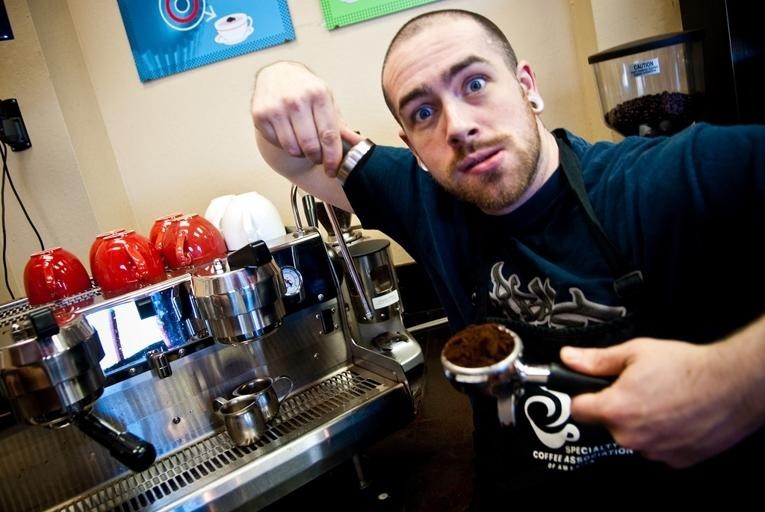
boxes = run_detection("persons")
[252,9,765,511]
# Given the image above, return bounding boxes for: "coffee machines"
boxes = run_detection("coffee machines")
[337,239,425,373]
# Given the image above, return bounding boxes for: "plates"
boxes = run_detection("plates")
[214,27,254,46]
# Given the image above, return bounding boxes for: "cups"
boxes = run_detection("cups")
[214,12,253,41]
[24,245,93,306]
[212,376,294,448]
[87,190,288,309]
